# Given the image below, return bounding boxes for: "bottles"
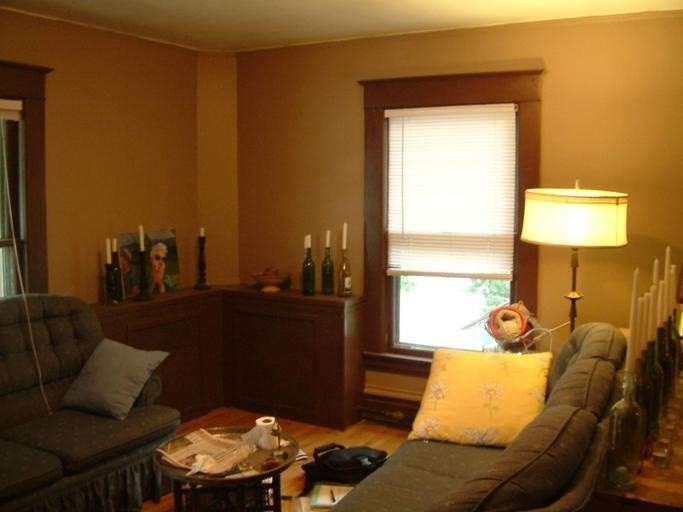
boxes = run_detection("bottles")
[609,369,643,491]
[104,264,115,305]
[638,307,679,460]
[111,252,121,303]
[321,247,334,295]
[302,248,314,296]
[337,249,352,297]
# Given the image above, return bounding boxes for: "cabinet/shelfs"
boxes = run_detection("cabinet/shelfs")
[86,283,225,426]
[221,283,366,431]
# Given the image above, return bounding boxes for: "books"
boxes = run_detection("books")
[310,482,354,508]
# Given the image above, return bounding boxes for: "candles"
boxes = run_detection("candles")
[112,238,118,253]
[625,242,678,369]
[324,229,330,248]
[199,227,204,237]
[304,232,312,249]
[105,237,112,264]
[341,222,347,250]
[138,224,145,251]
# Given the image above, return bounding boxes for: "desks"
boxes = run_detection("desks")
[613,376,683,511]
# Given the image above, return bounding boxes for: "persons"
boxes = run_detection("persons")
[147,240,178,295]
[107,246,140,299]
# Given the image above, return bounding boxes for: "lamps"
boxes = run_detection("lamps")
[520,179,629,337]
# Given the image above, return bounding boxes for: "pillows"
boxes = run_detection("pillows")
[408,348,554,446]
[62,336,171,420]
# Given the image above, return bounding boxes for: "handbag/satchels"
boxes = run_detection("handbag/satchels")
[303,444,388,484]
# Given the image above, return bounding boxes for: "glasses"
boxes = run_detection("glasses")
[152,255,167,262]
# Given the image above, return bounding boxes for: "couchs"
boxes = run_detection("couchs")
[0,293,182,510]
[321,320,627,512]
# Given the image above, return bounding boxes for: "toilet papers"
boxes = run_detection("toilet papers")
[256,415,279,451]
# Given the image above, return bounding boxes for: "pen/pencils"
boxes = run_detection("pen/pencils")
[330,489,336,502]
